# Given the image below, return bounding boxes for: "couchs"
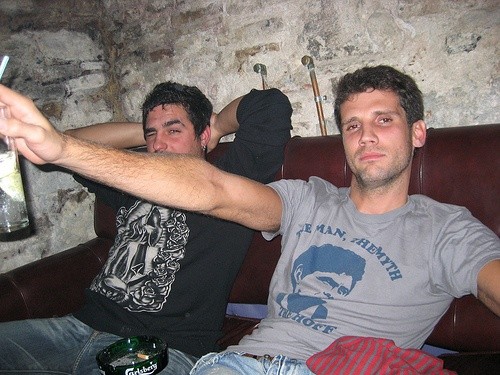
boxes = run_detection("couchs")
[1,123,499,375]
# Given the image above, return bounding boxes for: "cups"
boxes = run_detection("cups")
[0,146,29,233]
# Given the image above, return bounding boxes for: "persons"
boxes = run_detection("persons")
[0,80,294,375]
[0,64,500,374]
